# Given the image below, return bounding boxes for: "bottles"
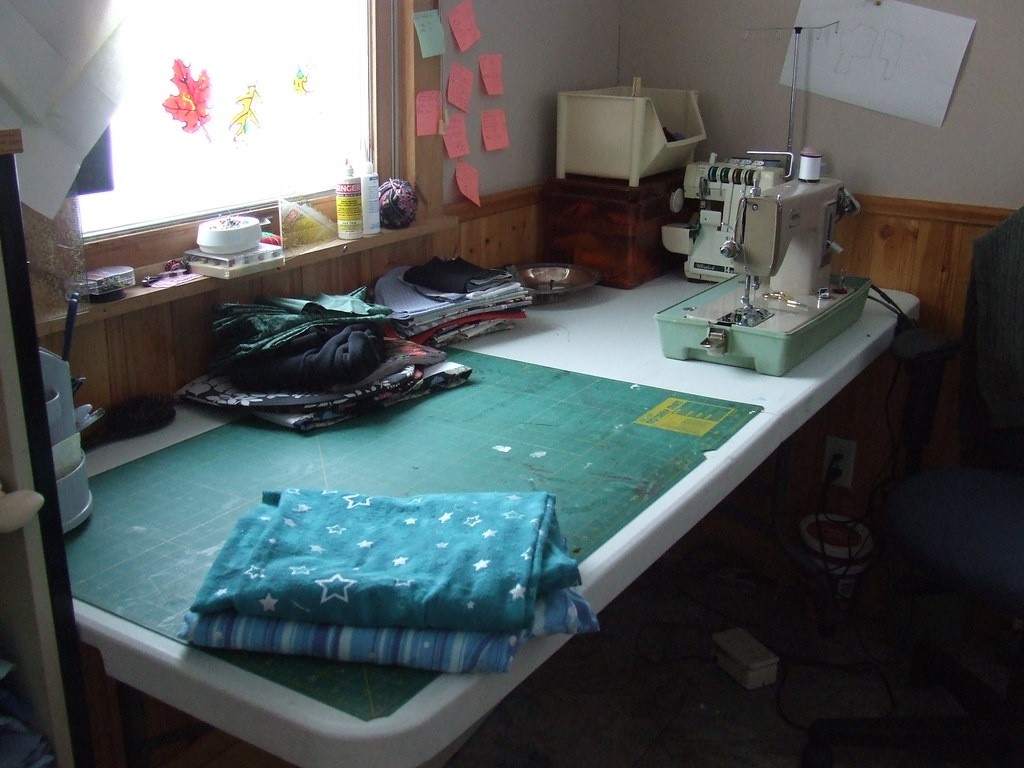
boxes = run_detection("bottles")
[336,155,364,239]
[359,155,380,234]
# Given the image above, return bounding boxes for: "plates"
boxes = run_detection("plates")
[513,264,602,304]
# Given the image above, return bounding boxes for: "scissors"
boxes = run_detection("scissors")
[62,290,79,361]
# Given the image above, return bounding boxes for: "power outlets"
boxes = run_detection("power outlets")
[822,436,856,490]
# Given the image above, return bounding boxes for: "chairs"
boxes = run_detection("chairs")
[800,206,1024,768]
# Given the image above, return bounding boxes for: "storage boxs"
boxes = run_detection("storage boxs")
[538,84,707,291]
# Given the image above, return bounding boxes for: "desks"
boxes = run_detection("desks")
[64,265,921,768]
